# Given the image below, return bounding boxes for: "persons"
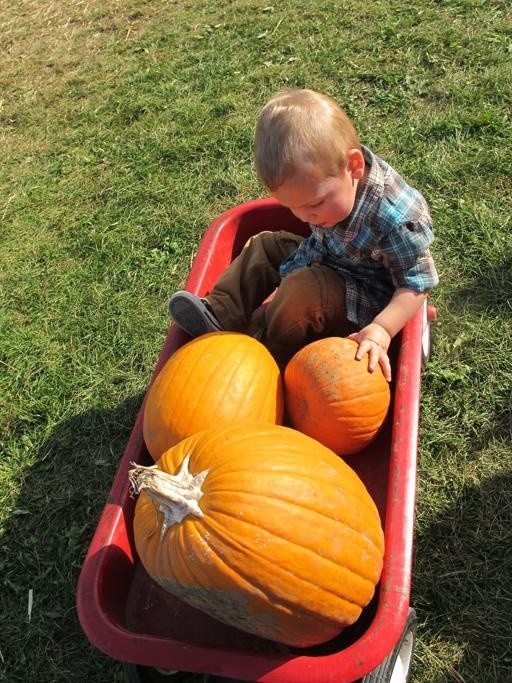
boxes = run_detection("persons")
[169,89,439,381]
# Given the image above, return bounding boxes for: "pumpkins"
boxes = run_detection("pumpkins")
[129,422,386,649]
[283,335,392,455]
[144,331,284,462]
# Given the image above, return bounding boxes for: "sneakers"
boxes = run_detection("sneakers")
[168,290,225,339]
